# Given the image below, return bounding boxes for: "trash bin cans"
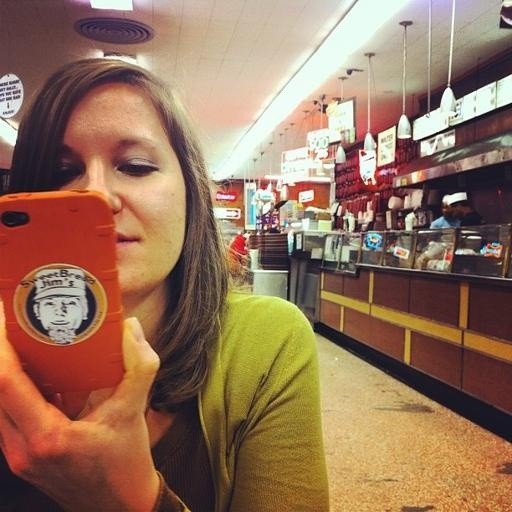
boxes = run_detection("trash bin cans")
[249,270,288,301]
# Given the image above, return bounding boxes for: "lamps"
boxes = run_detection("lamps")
[249,112,297,205]
[335,76,347,163]
[364,52,377,153]
[440,0,459,116]
[397,19,413,139]
[307,93,331,162]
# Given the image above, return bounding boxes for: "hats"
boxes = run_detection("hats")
[442,192,467,205]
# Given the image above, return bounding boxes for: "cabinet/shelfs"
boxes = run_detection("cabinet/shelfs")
[320,222,512,286]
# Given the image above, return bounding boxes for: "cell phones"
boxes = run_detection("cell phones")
[0,190,126,420]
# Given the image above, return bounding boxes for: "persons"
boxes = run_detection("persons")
[0,59,329,512]
[429,192,483,230]
